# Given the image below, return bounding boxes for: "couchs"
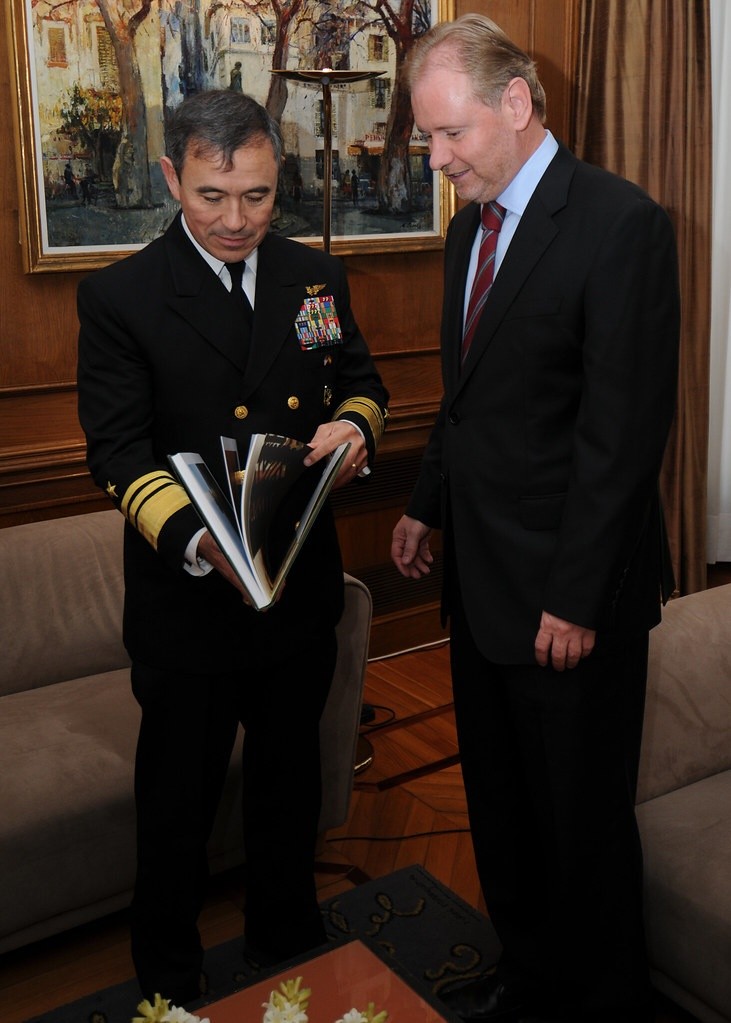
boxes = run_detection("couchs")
[0,499,374,973]
[634,582,731,1023]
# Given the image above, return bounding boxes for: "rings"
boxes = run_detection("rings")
[352,462,358,473]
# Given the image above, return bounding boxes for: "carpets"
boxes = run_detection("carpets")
[24,864,494,1023]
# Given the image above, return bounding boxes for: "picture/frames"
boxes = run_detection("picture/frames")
[3,0,457,279]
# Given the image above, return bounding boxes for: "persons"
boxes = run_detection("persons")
[75,91,390,1015]
[391,12,680,991]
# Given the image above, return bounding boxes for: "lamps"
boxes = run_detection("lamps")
[273,66,388,776]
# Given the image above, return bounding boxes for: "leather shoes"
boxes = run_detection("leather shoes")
[454,975,506,1021]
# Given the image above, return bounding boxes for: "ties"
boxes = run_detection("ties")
[459,201,507,374]
[224,260,253,311]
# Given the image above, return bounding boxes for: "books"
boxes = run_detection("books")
[166,433,352,613]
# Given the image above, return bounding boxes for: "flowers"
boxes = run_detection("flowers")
[260,976,311,1023]
[130,990,210,1023]
[336,1002,389,1023]
